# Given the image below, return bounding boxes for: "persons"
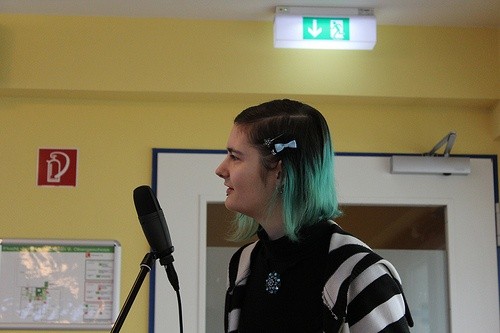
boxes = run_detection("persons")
[213,97,414,333]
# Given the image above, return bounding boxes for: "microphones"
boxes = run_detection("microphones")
[132,185,180,292]
